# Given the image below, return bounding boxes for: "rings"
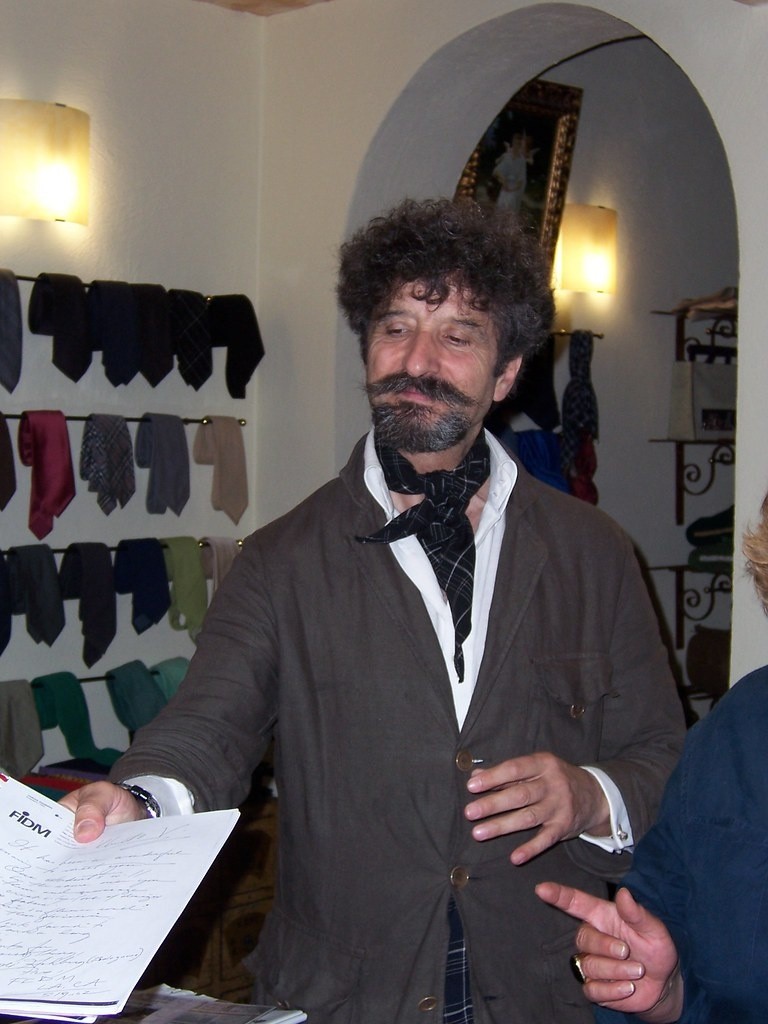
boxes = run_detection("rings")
[570,954,589,985]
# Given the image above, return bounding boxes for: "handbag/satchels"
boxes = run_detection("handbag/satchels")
[663,345,736,442]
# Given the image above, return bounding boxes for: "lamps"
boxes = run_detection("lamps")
[548,202,618,292]
[0,97,91,225]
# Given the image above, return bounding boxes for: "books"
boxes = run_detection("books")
[0,772,242,1020]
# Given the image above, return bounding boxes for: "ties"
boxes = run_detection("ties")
[0,268,271,779]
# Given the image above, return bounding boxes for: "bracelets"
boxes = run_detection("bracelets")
[115,781,162,822]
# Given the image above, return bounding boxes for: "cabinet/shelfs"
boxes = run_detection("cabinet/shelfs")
[648,286,738,731]
[132,796,281,1003]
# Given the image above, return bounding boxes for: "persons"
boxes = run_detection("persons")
[61,196,687,1023]
[534,497,768,1022]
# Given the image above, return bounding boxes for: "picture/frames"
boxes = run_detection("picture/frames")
[452,77,584,276]
